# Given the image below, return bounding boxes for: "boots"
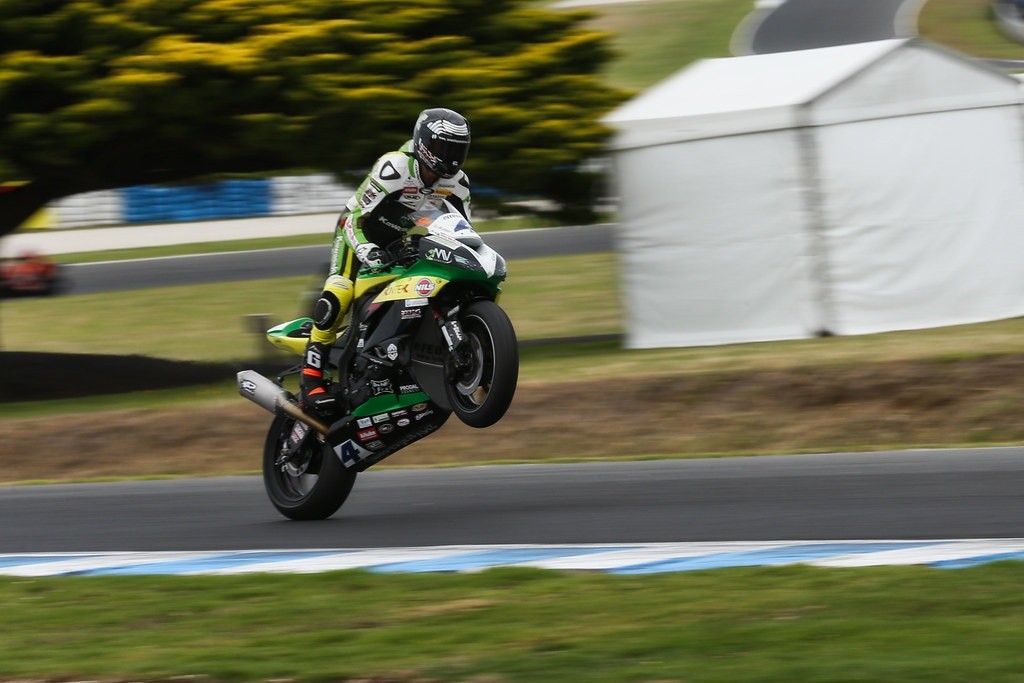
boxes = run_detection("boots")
[299,342,335,409]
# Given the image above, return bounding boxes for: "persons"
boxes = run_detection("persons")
[300,108,472,407]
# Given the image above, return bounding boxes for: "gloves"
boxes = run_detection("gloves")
[364,248,395,273]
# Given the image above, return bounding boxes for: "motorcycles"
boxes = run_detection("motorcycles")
[232,197,519,522]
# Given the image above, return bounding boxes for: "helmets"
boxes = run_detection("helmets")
[413,108,471,179]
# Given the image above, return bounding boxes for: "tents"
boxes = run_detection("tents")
[597,35,1024,350]
